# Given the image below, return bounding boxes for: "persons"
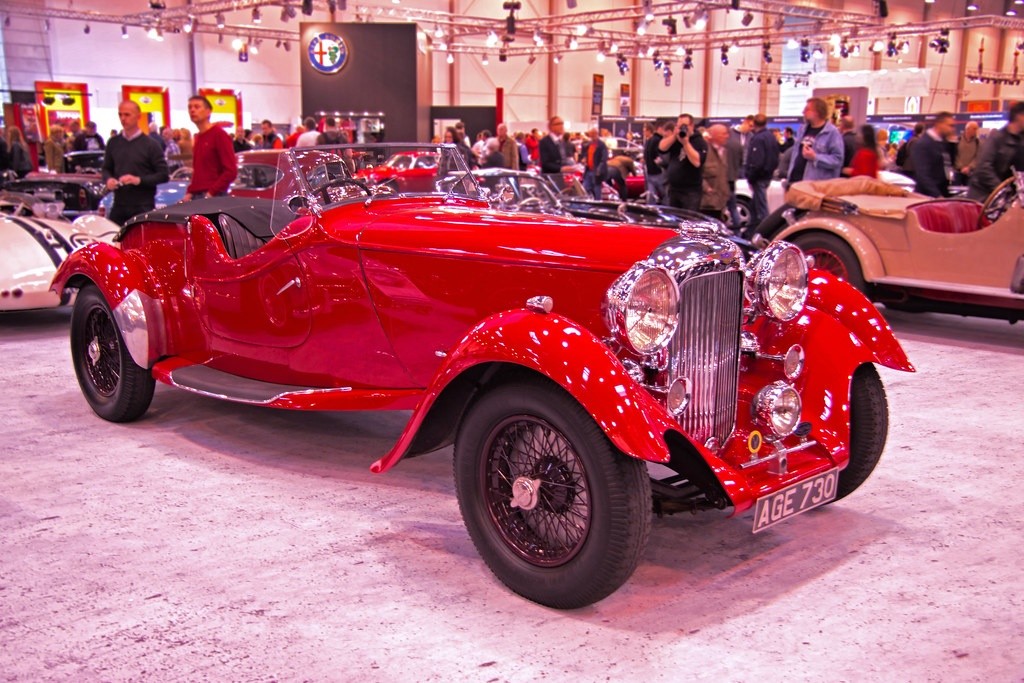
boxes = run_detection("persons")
[286,114,353,160]
[228,119,284,152]
[41,119,106,174]
[727,113,798,235]
[102,100,171,226]
[966,96,1024,203]
[179,95,239,200]
[904,124,924,171]
[945,121,999,185]
[910,110,957,197]
[838,114,905,179]
[642,113,743,233]
[786,98,846,187]
[442,121,532,185]
[6,126,33,179]
[0,127,13,172]
[147,122,194,174]
[527,113,638,202]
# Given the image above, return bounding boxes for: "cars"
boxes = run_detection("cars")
[524,136,648,201]
[719,169,916,238]
[225,147,361,209]
[348,151,441,195]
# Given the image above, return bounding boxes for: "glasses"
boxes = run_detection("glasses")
[554,122,564,126]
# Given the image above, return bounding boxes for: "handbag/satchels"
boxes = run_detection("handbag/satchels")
[895,142,908,166]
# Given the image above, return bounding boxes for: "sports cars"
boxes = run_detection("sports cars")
[50,143,916,611]
[1,150,193,314]
[766,166,1024,325]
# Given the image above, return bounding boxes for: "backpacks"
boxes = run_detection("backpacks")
[322,131,343,159]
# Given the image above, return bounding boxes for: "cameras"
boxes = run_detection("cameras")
[116,181,124,186]
[678,123,688,138]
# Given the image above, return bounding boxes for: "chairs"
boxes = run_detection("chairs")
[219,202,295,257]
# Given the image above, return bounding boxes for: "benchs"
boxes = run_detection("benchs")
[355,0,951,87]
[906,197,992,234]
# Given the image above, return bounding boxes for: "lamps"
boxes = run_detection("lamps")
[302,0,313,15]
[967,70,1022,86]
[215,12,224,30]
[281,1,295,21]
[327,0,347,14]
[252,6,263,26]
[184,16,193,32]
[247,37,262,55]
[121,24,129,39]
[275,39,292,52]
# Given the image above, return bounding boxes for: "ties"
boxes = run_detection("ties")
[558,138,562,146]
